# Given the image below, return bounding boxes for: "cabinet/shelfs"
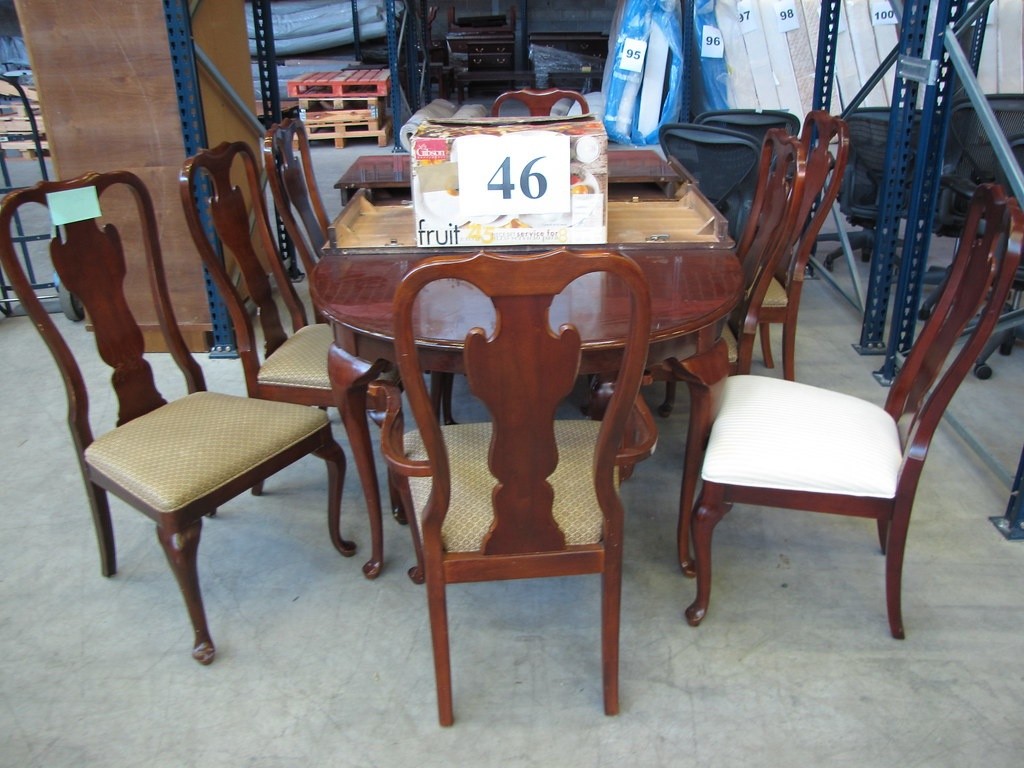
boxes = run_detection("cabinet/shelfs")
[445,32,609,71]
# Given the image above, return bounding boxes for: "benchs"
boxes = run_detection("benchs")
[455,71,605,105]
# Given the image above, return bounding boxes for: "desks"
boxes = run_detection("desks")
[305,149,751,583]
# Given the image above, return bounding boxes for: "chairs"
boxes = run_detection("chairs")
[582,125,807,419]
[364,240,662,729]
[263,117,459,425]
[745,108,850,381]
[677,183,1024,639]
[490,84,589,118]
[657,90,1024,381]
[175,137,445,528]
[0,170,358,667]
